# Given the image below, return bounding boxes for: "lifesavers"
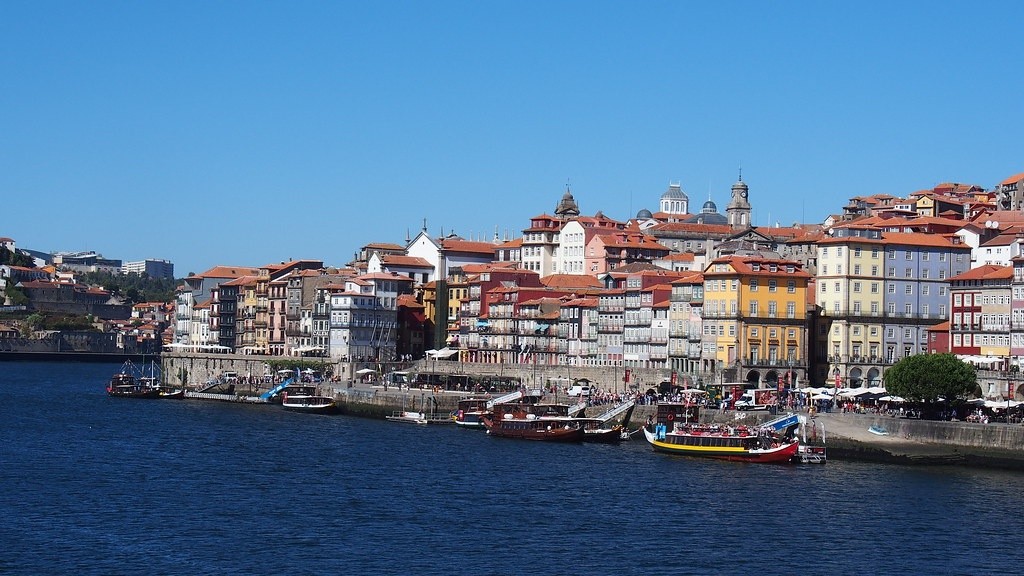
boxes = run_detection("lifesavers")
[668,414,674,421]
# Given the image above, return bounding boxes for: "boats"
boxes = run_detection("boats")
[867,425,890,436]
[278,381,336,411]
[386,410,452,425]
[158,389,186,399]
[643,400,826,466]
[452,388,636,441]
[104,357,162,398]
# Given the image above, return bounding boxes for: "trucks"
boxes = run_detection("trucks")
[735,389,779,412]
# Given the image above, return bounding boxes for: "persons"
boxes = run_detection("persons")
[941,409,1024,425]
[365,374,521,393]
[238,373,338,384]
[792,398,922,419]
[543,385,727,413]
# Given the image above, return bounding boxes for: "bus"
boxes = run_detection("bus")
[223,371,239,383]
[705,383,752,409]
[277,368,321,383]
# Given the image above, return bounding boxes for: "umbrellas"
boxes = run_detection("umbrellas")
[966,397,1024,409]
[794,386,944,402]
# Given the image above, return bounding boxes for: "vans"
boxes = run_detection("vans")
[566,386,590,396]
